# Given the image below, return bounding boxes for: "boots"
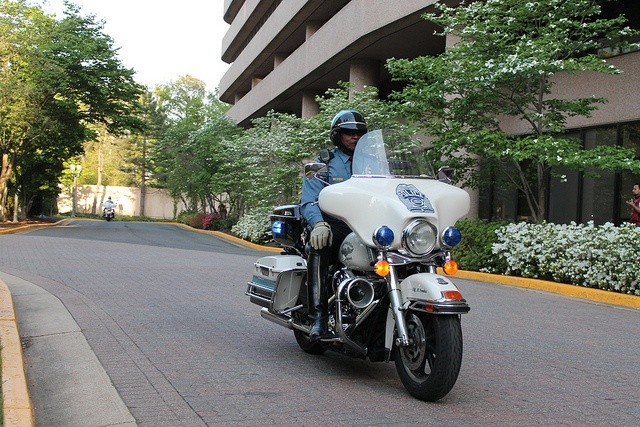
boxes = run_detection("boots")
[308,254,329,338]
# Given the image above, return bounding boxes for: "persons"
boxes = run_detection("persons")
[301,110,388,338]
[103,196,115,217]
[626,184,640,227]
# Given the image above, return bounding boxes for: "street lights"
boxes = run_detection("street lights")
[70,164,82,218]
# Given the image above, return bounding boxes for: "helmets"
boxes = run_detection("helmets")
[330,110,368,141]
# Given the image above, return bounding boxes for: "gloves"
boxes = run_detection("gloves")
[310,221,332,250]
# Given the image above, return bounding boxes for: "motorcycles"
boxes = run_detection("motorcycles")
[245,129,471,402]
[100,201,117,222]
